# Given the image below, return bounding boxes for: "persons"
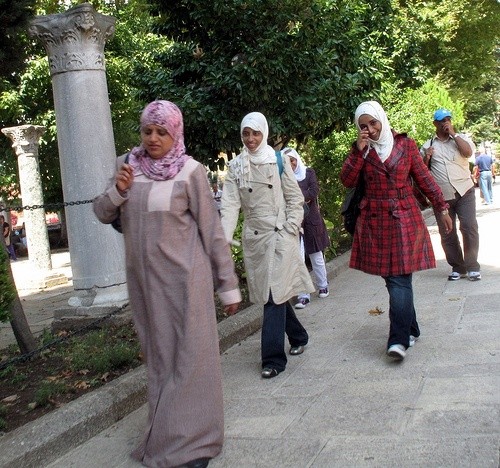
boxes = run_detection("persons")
[0,215,17,260]
[339,101,453,360]
[473,146,495,205]
[93,100,244,468]
[279,147,331,309]
[18,222,27,249]
[220,112,316,378]
[208,183,222,218]
[419,109,481,281]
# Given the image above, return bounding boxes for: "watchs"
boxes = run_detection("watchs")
[453,133,459,139]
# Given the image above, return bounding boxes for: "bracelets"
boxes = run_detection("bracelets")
[437,208,449,216]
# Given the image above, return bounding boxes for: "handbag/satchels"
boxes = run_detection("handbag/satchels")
[108,153,131,232]
[342,166,364,236]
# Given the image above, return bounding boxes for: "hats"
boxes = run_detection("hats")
[434,108,451,118]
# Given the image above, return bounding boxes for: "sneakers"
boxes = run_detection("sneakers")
[448,271,481,282]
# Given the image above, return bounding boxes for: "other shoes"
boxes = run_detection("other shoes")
[187,457,210,468]
[262,368,277,378]
[318,288,329,298]
[289,345,304,353]
[388,334,418,359]
[295,296,310,309]
